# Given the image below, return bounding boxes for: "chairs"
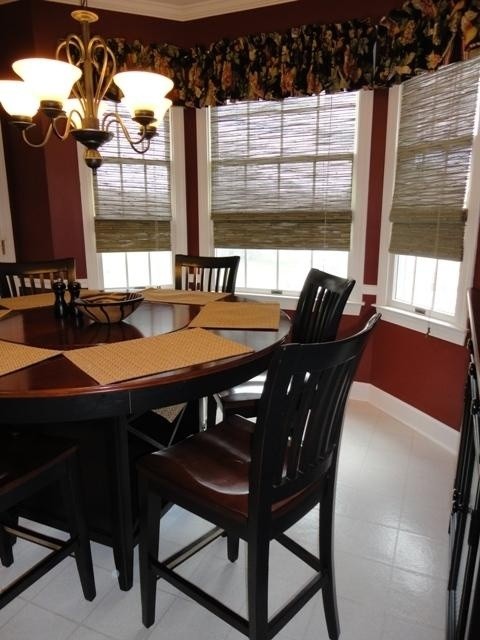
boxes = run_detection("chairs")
[126,306,387,639]
[198,266,363,544]
[171,248,242,295]
[0,256,78,303]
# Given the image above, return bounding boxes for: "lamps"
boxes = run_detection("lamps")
[0,0,178,180]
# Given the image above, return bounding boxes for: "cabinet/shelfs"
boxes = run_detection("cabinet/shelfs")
[442,283,480,640]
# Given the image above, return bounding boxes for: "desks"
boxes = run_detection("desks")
[0,287,297,597]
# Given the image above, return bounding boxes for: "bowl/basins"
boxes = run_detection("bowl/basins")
[81,322,144,347]
[73,292,144,324]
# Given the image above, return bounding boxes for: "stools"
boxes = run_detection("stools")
[0,420,102,614]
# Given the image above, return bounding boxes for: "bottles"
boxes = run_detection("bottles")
[67,282,84,316]
[53,281,68,316]
